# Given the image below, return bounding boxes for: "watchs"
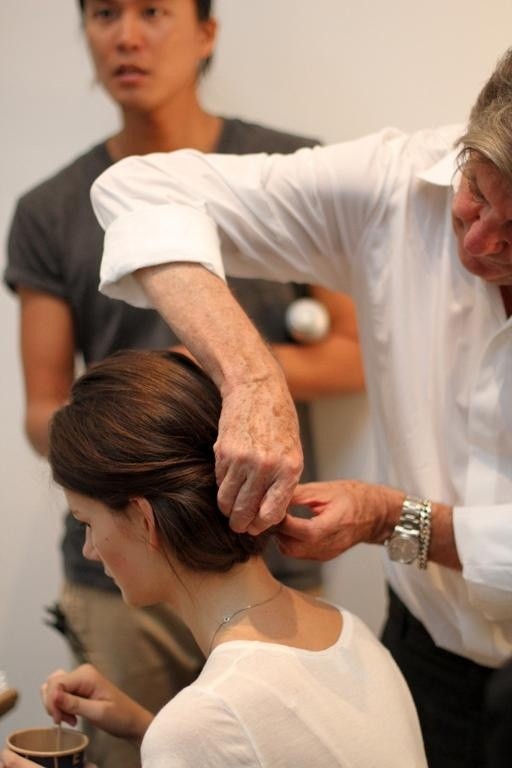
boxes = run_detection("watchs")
[384,495,424,565]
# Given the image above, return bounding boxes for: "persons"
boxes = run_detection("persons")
[0,351,427,768]
[3,0,366,768]
[90,46,512,768]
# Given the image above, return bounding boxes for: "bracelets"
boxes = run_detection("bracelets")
[417,499,432,570]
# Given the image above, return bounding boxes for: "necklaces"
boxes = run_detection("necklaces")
[208,583,283,654]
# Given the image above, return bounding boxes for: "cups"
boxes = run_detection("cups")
[9,727,91,768]
